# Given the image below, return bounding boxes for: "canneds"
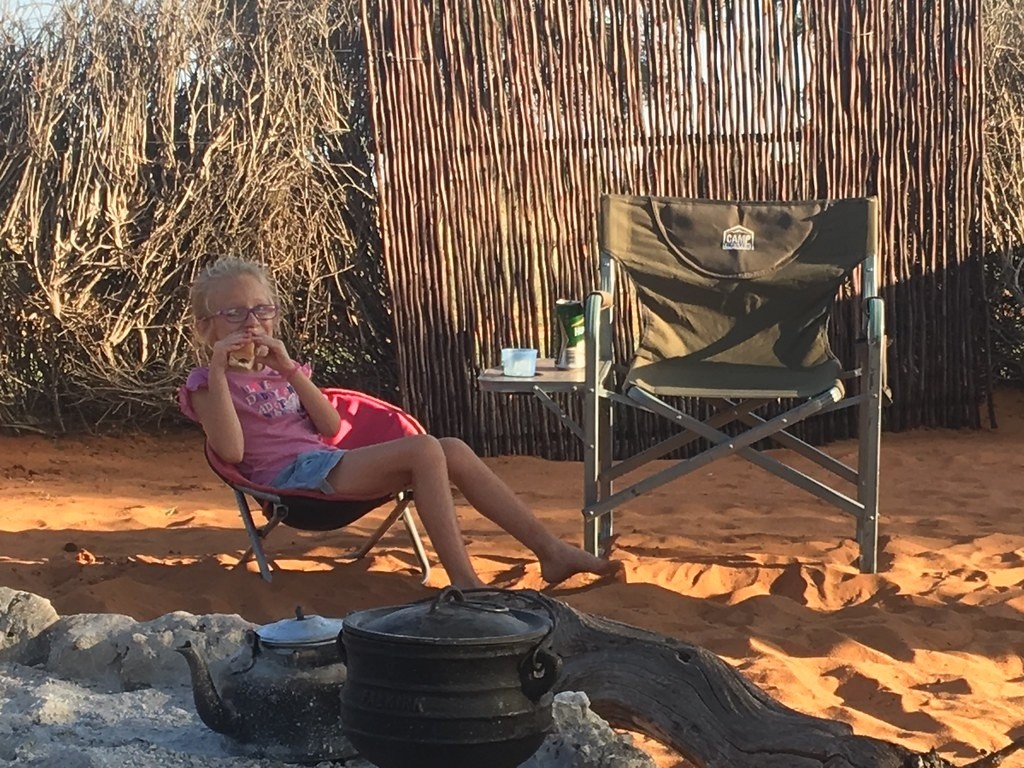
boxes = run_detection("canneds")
[553,297,586,371]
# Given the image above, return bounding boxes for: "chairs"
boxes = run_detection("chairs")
[587,198,893,580]
[203,388,430,584]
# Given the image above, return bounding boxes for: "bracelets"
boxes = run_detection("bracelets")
[284,360,300,379]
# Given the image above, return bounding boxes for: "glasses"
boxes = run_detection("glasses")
[200,303,279,324]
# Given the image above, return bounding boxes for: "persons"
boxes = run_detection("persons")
[180,259,624,592]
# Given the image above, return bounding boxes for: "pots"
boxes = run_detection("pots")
[337,586,565,768]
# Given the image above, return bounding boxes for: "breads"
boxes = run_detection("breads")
[228,342,255,369]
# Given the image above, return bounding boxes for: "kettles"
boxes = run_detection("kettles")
[174,607,359,759]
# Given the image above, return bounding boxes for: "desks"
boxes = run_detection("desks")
[480,359,610,445]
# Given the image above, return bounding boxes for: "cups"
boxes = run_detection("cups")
[502,349,538,376]
